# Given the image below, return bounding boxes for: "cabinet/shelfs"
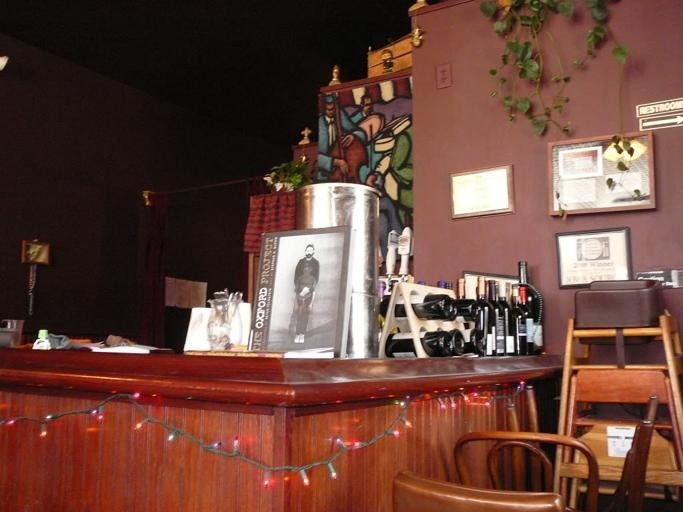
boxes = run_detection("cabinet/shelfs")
[1,350,562,512]
[552,281,683,509]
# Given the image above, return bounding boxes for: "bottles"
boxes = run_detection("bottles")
[455,278,471,354]
[378,293,476,322]
[475,275,496,356]
[384,328,486,358]
[31,329,55,351]
[497,280,510,356]
[518,260,545,354]
[508,283,527,356]
[486,279,506,356]
[519,286,534,355]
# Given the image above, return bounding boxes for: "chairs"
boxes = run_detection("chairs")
[394,429,603,512]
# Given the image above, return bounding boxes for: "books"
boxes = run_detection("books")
[84,339,175,355]
[246,223,351,355]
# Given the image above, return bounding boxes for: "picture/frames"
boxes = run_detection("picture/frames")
[447,131,658,289]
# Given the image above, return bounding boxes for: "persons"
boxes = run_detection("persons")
[296,265,315,312]
[293,244,320,344]
[94,334,137,346]
